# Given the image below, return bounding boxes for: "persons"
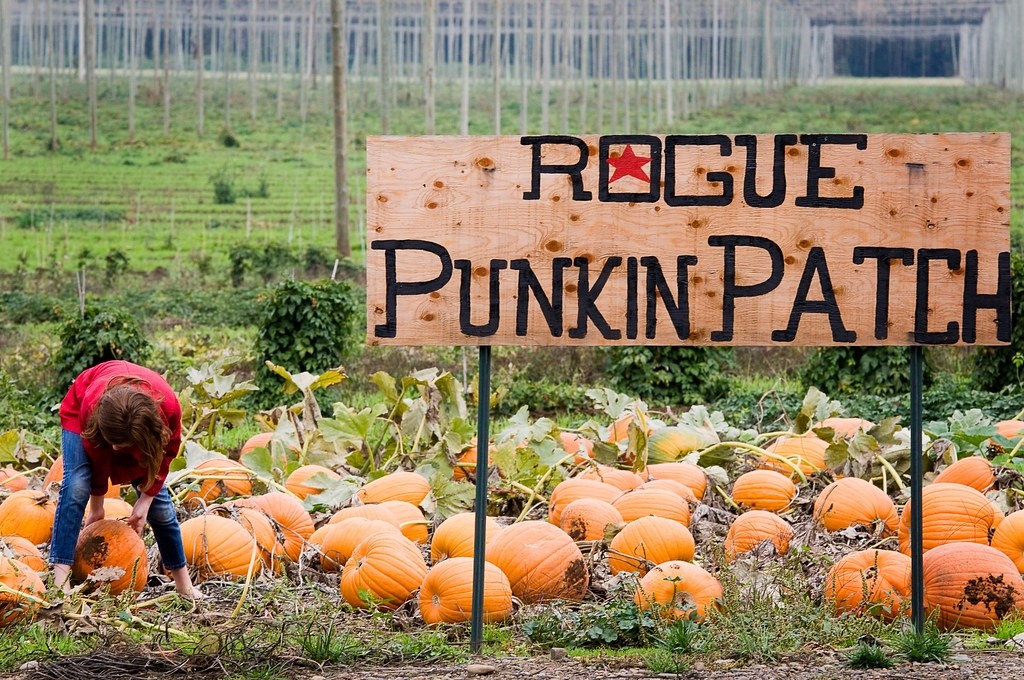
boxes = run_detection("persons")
[48,360,211,598]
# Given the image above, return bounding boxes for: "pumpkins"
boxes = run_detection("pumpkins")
[0,412,1024,634]
